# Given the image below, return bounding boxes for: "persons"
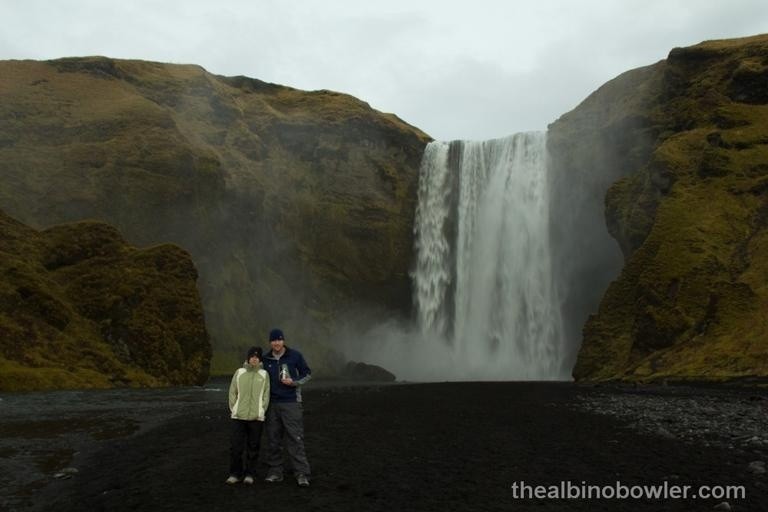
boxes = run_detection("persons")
[260,328,313,486]
[226,345,270,486]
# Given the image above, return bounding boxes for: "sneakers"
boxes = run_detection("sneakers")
[226,476,239,484]
[298,477,310,486]
[244,477,253,484]
[265,475,284,482]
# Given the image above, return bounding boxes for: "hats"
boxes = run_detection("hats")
[248,346,263,364]
[269,329,284,341]
